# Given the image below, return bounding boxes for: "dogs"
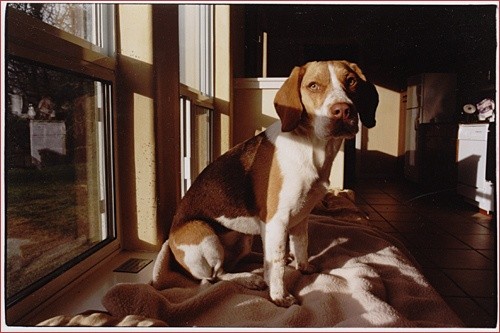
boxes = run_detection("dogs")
[168,59,380,308]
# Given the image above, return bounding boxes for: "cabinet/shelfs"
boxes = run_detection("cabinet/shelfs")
[233,77,344,191]
[457,123,495,214]
